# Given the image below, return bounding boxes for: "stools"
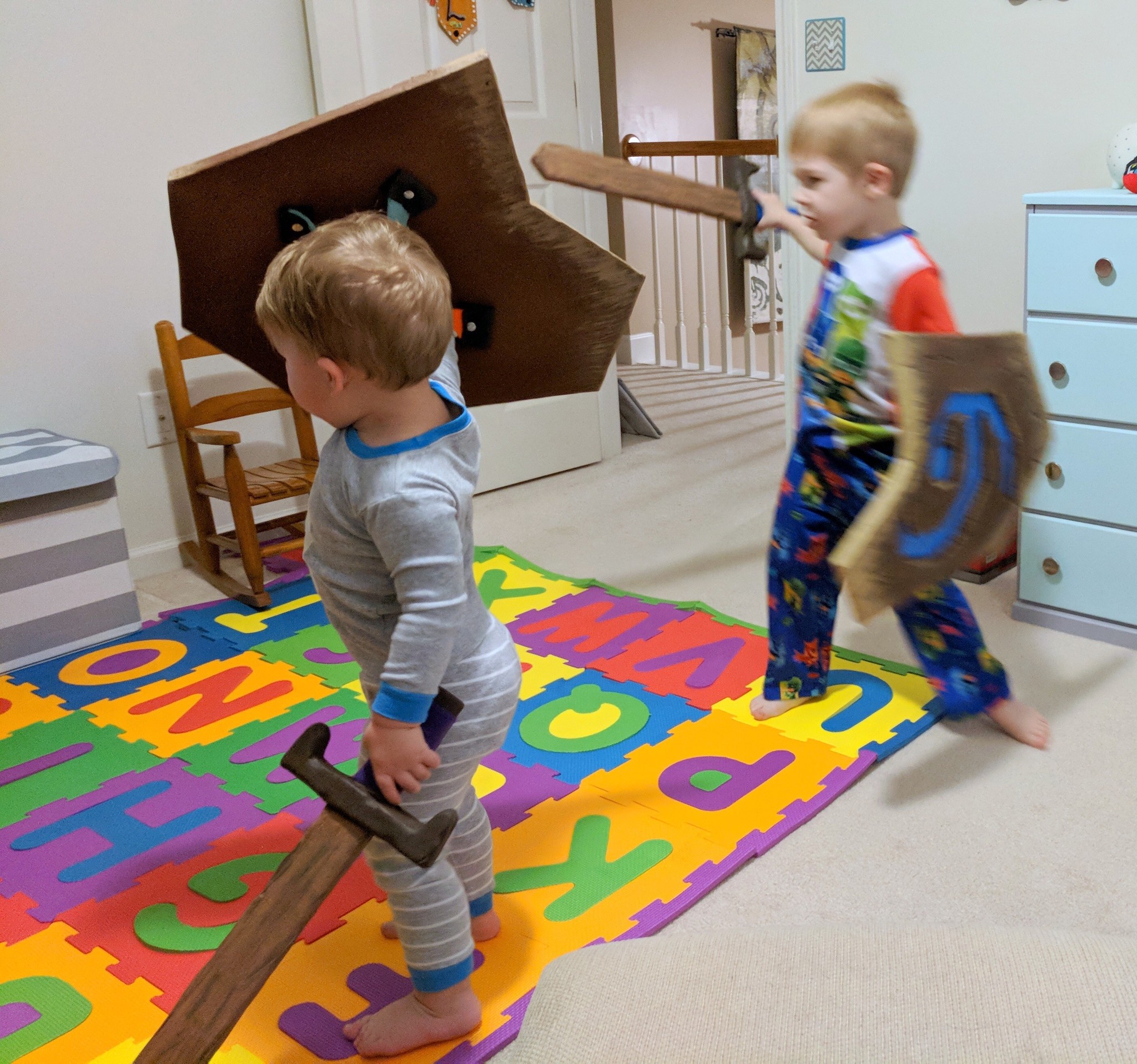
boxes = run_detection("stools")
[0,425,146,676]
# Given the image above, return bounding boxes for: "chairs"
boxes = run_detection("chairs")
[151,319,322,611]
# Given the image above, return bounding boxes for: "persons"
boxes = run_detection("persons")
[749,75,1051,751]
[255,210,523,1058]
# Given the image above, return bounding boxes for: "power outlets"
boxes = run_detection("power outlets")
[140,383,196,448]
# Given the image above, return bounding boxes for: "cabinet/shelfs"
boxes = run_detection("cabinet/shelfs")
[1009,186,1137,650]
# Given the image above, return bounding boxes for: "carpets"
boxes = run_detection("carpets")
[0,539,946,1064]
